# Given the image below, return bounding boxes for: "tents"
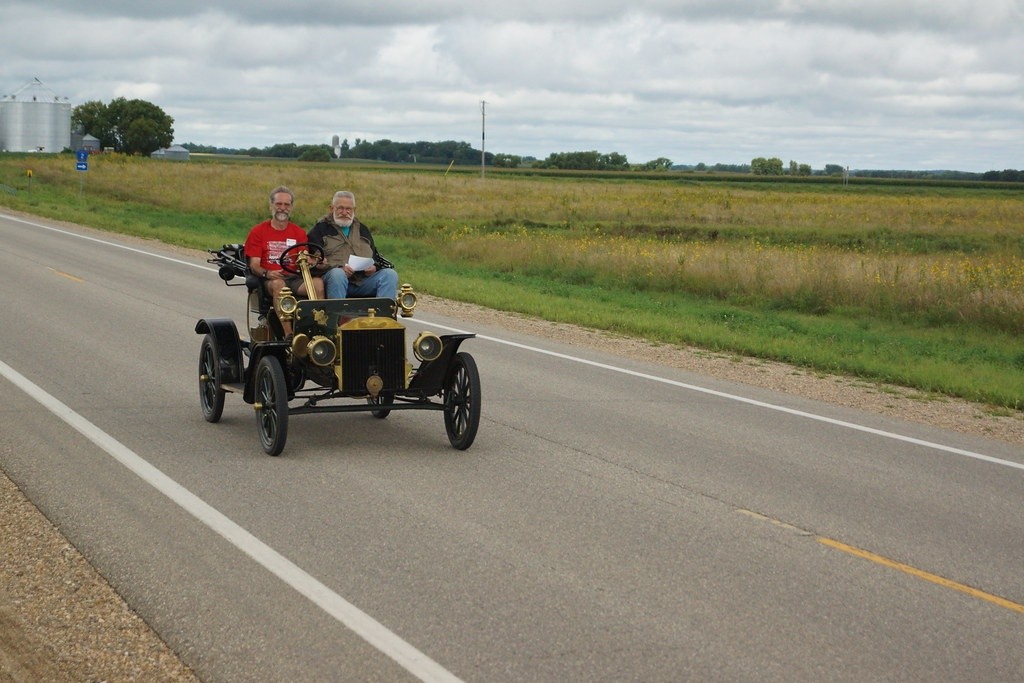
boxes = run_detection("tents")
[150,145,189,161]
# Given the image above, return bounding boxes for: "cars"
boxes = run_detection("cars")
[195,239,481,457]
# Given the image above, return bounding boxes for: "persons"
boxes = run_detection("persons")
[244,187,327,346]
[306,191,399,344]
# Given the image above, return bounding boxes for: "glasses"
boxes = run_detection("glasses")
[333,205,355,212]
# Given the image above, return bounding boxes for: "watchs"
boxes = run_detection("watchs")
[262,271,267,280]
